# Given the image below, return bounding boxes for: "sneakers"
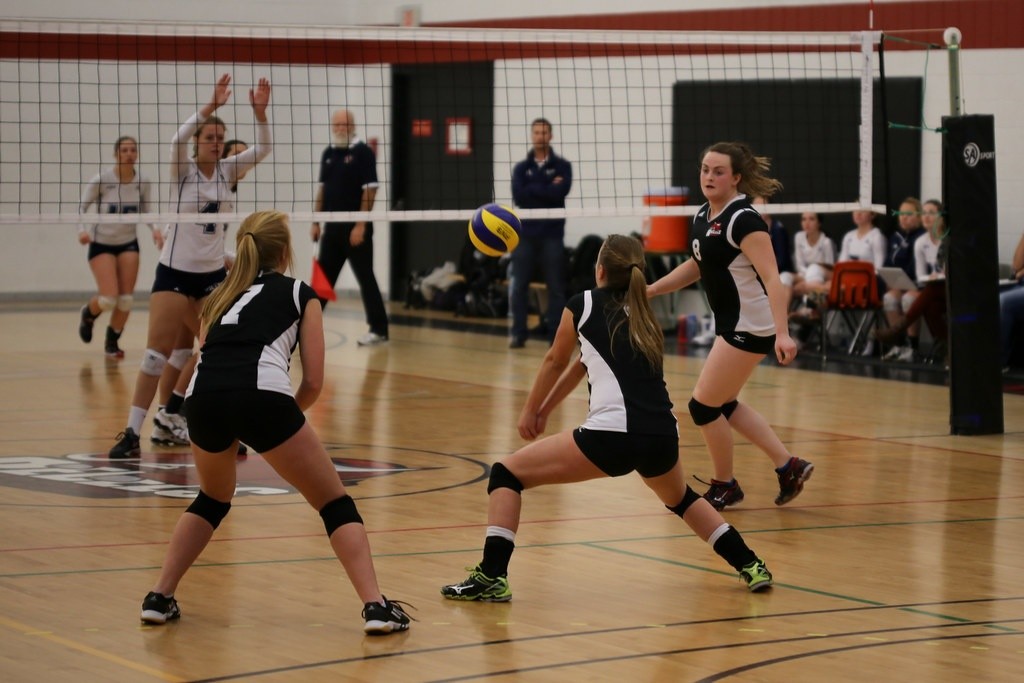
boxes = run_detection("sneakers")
[140,591,180,623]
[79,303,94,343]
[441,565,512,603]
[774,453,814,506]
[739,559,773,590]
[237,443,246,456]
[150,421,188,445]
[362,592,417,633]
[105,340,124,356]
[153,406,191,442]
[109,428,140,458]
[693,474,744,512]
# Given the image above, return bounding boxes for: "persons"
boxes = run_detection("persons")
[140,209,419,639]
[76,136,164,356]
[1000,230,1024,374]
[507,117,574,348]
[307,110,391,346]
[748,195,946,367]
[647,142,814,508]
[440,234,774,601]
[105,74,274,457]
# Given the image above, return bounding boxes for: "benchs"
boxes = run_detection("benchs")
[446,273,547,319]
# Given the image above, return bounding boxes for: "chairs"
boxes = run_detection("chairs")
[636,194,711,314]
[788,260,946,365]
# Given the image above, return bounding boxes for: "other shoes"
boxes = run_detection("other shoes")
[861,340,875,356]
[358,331,387,346]
[693,332,713,345]
[881,346,901,362]
[509,335,523,348]
[899,347,917,364]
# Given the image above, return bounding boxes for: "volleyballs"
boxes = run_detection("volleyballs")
[467,200,523,258]
